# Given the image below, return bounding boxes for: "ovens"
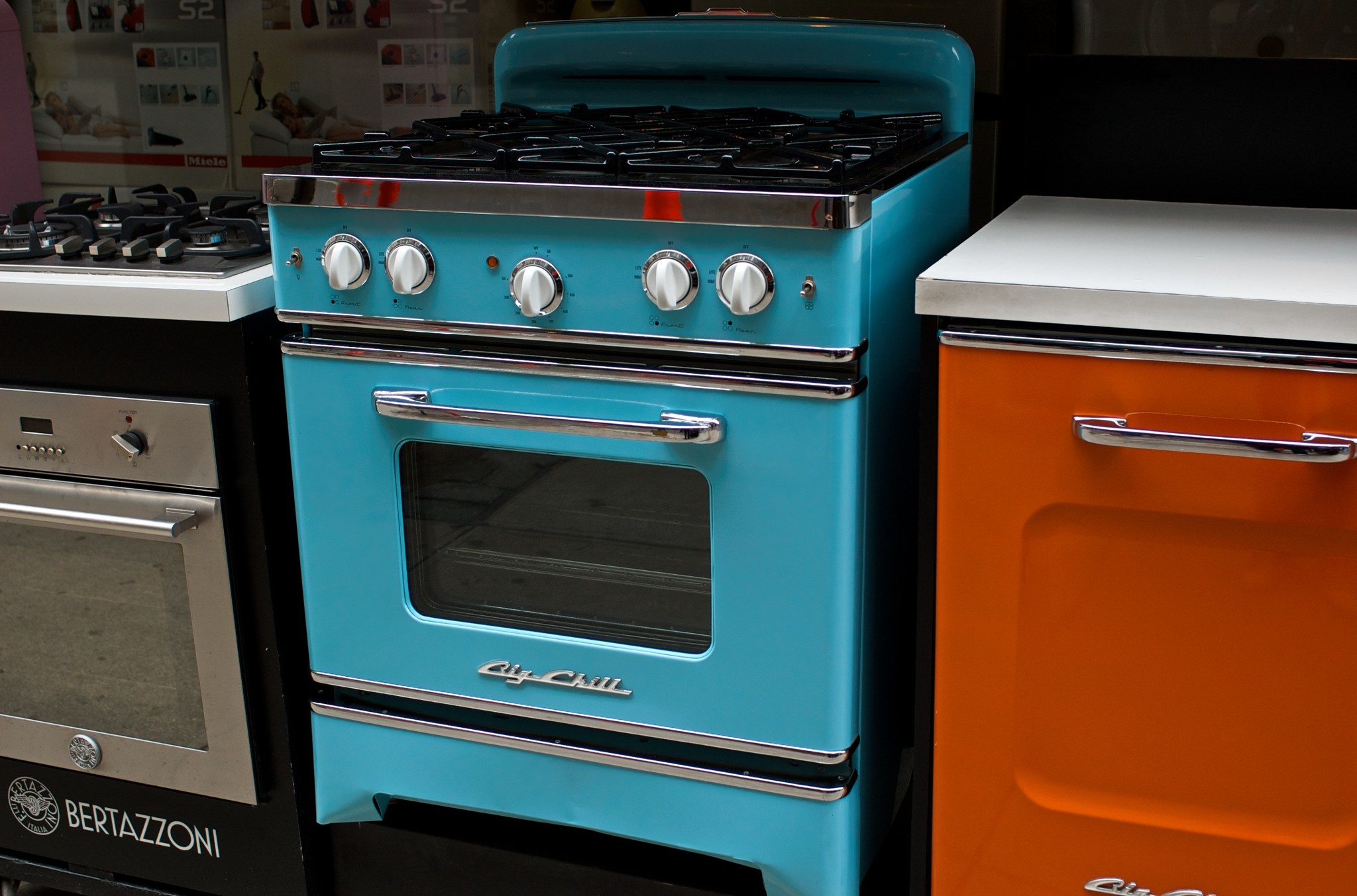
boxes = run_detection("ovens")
[1,305,865,893]
[933,320,1357,896]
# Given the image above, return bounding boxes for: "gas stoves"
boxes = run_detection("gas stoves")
[262,16,975,228]
[1,182,276,322]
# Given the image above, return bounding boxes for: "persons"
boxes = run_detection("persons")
[45,90,140,134]
[273,91,413,136]
[46,106,131,139]
[24,52,42,107]
[248,49,268,110]
[272,109,363,140]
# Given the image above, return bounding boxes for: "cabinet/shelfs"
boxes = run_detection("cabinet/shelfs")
[928,315,1357,895]
